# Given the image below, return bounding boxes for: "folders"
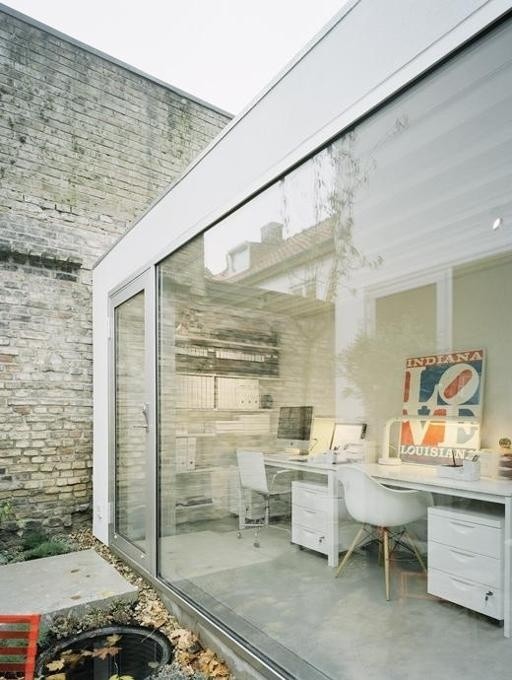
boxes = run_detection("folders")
[238,379,260,410]
[176,438,196,472]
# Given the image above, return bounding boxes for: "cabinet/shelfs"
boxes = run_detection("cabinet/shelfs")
[176,407,282,438]
[229,464,291,521]
[291,478,373,559]
[425,498,504,627]
[175,333,282,381]
[175,464,227,525]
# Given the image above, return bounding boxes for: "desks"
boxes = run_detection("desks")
[336,457,511,637]
[243,443,380,569]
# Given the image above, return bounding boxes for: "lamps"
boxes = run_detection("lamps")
[377,415,483,465]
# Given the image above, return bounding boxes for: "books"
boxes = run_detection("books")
[285,446,309,455]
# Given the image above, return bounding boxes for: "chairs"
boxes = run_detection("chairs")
[235,448,292,548]
[334,465,436,603]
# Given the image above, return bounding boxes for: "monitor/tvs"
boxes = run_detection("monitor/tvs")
[275,406,315,461]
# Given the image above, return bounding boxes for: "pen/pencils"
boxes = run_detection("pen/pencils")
[472,454,480,462]
[332,444,341,464]
